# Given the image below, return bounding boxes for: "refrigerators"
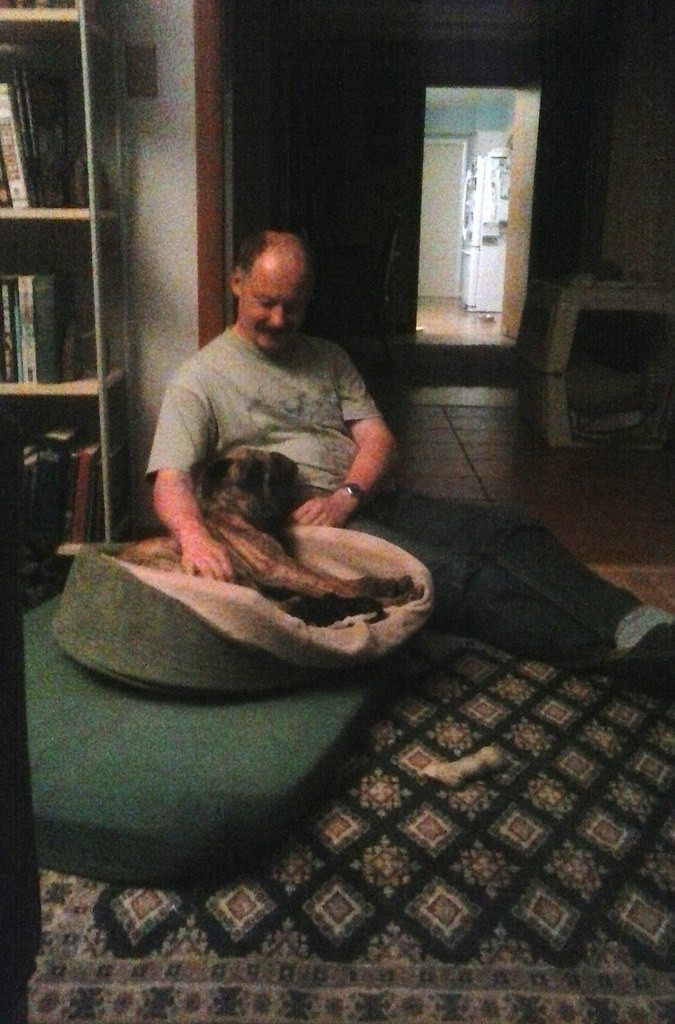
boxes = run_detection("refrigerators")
[458,151,509,313]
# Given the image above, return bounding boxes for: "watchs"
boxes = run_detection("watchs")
[338,482,364,509]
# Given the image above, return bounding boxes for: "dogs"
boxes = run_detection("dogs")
[110,447,425,623]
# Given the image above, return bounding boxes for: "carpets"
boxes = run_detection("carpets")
[0,577,675,1024]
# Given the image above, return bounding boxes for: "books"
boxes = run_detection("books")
[21,427,131,547]
[0,58,67,209]
[0,272,80,383]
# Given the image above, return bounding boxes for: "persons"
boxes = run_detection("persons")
[143,230,675,695]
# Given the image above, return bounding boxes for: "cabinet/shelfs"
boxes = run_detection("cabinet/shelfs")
[1,1,137,577]
